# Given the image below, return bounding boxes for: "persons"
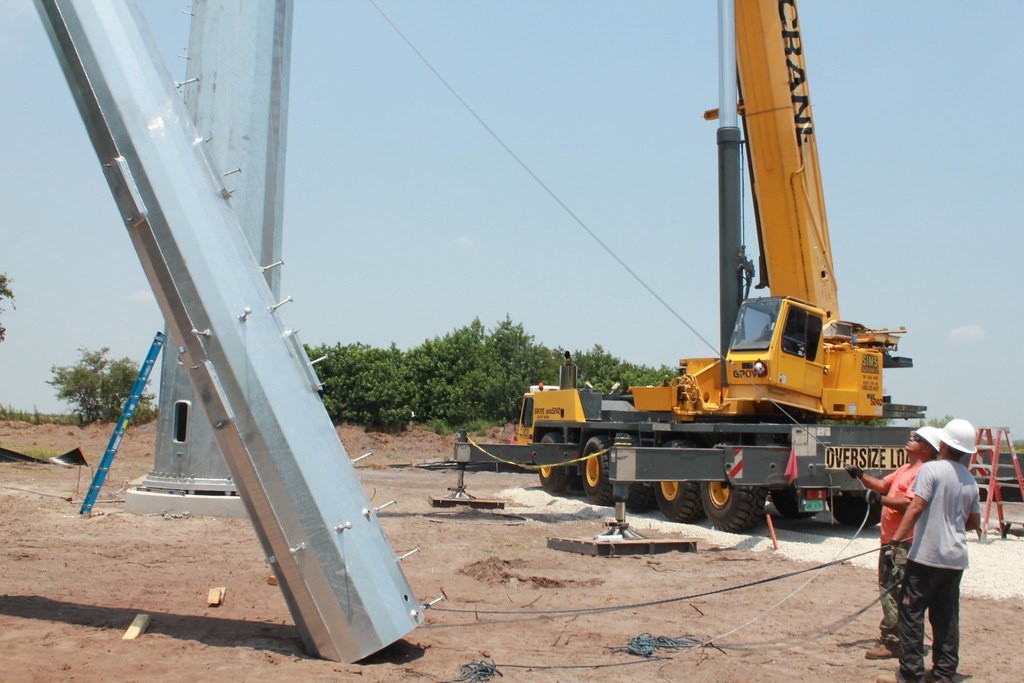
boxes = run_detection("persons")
[845,426,940,659]
[875,419,981,683]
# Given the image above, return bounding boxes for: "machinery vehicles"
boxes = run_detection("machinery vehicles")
[421,0,940,560]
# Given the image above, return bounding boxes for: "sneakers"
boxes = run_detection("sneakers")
[865,643,897,660]
[875,670,914,683]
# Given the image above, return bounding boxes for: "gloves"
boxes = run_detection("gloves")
[845,464,864,479]
[883,539,899,569]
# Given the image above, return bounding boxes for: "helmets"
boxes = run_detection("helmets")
[939,419,977,454]
[910,425,940,452]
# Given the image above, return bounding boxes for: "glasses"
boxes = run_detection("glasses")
[910,434,929,448]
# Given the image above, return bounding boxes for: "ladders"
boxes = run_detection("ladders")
[76,329,166,519]
[965,426,1024,545]
